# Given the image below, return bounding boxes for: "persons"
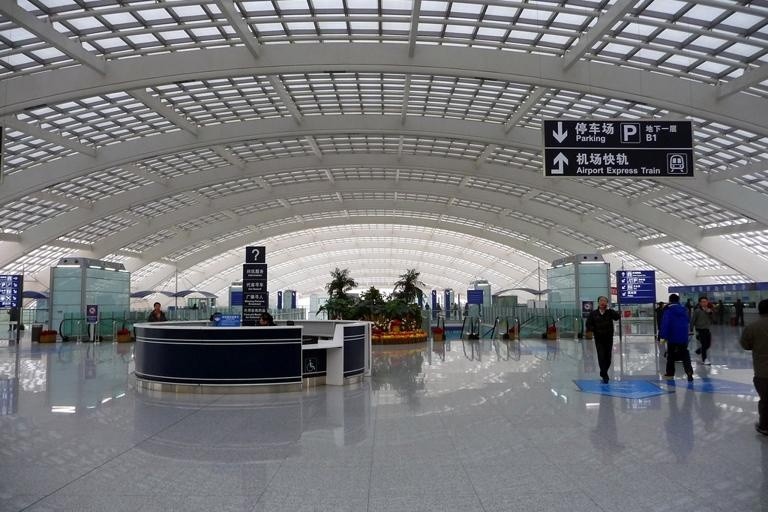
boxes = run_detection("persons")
[191,304,197,310]
[740,297,768,436]
[585,295,621,385]
[256,312,277,326]
[654,297,746,342]
[425,302,429,311]
[689,295,716,365]
[147,301,167,323]
[436,302,442,313]
[7,306,18,332]
[660,294,694,383]
[464,303,469,316]
[450,303,455,313]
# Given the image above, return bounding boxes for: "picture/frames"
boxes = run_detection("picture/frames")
[508,334,520,340]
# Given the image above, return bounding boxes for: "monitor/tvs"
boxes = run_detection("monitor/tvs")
[221,314,241,327]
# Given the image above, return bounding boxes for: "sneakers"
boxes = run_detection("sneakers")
[599,371,610,384]
[755,421,768,437]
[662,358,711,381]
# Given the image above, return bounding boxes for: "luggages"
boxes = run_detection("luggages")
[730,317,738,327]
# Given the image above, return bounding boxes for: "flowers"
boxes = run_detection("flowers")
[116,326,128,337]
[546,325,556,332]
[37,329,56,337]
[430,326,443,334]
[506,327,520,333]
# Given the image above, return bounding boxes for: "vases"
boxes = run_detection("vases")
[433,334,441,342]
[547,333,556,340]
[39,336,55,343]
[117,333,131,342]
